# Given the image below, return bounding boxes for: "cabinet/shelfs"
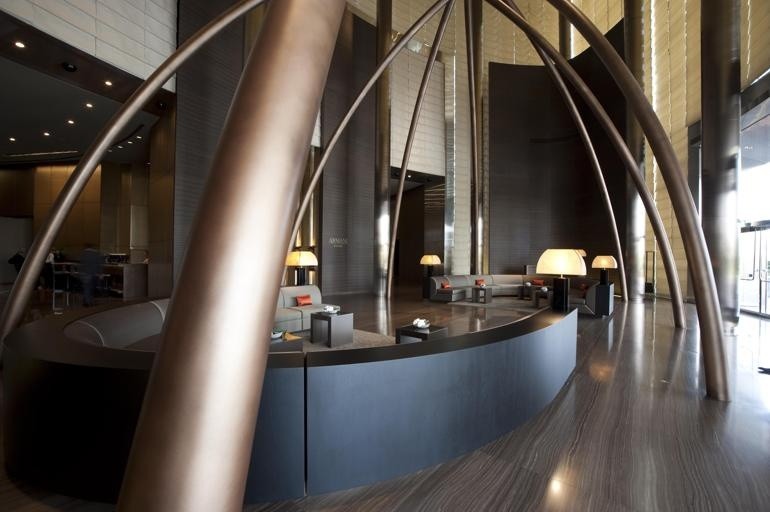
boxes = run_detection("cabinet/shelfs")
[422,277,430,299]
[35,263,147,301]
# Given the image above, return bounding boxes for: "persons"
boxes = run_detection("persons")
[8,246,25,274]
[77,247,105,307]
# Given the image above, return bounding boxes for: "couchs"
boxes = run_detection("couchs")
[2,284,578,499]
[430,275,615,317]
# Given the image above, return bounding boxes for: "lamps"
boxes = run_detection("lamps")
[286,251,319,285]
[536,249,618,315]
[420,255,441,278]
